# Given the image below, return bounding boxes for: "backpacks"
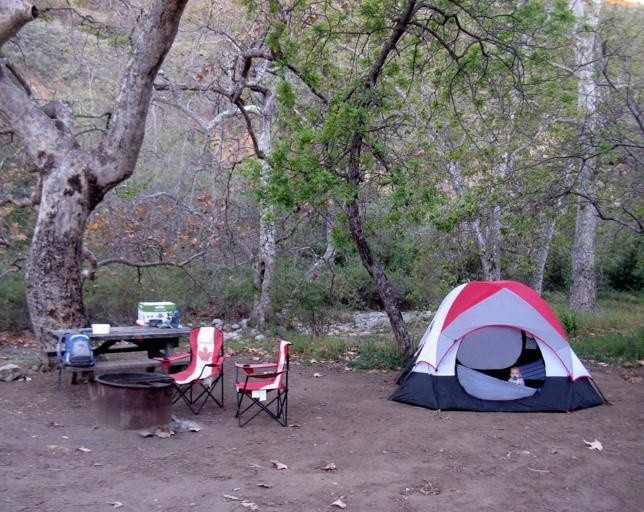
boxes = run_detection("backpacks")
[62,333,94,366]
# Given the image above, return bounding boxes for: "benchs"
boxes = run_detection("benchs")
[39,340,180,401]
[69,353,192,386]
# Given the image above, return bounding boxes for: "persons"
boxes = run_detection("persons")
[508,366,525,388]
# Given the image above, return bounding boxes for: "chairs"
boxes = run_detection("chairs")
[235,339,293,428]
[154,324,225,415]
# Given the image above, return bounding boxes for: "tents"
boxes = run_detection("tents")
[386,279,608,412]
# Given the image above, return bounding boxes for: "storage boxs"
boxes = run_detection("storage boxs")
[91,324,110,334]
[138,302,176,325]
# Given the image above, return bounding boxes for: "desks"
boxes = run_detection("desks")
[49,326,193,390]
